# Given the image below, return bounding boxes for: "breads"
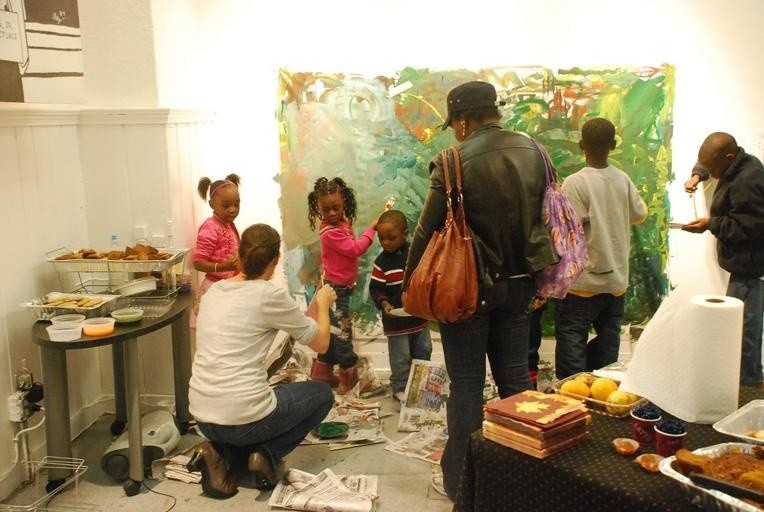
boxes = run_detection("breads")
[34,294,104,309]
[56,240,174,262]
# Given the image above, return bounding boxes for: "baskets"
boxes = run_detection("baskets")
[553,371,649,418]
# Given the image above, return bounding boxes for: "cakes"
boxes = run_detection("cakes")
[632,453,665,472]
[612,436,640,456]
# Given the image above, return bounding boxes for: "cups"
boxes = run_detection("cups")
[655,426,687,455]
[630,409,662,444]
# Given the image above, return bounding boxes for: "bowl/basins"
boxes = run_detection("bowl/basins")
[82,318,115,336]
[51,314,85,324]
[111,309,144,322]
[46,324,85,340]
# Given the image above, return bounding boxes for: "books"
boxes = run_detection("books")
[163,444,203,486]
[479,389,591,459]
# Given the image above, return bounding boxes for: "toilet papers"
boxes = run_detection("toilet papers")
[618,294,744,426]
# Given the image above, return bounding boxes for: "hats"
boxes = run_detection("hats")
[442,82,499,130]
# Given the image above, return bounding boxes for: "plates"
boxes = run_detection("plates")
[391,308,413,317]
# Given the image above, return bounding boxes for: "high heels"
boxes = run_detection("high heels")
[187,440,239,497]
[248,452,277,489]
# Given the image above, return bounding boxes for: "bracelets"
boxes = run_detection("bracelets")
[214,263,217,274]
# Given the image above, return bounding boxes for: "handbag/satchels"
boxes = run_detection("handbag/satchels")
[536,182,588,300]
[403,202,479,326]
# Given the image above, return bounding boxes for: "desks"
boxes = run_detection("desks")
[459,360,764,510]
[30,289,195,499]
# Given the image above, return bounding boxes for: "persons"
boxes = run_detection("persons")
[679,131,764,387]
[190,172,242,318]
[401,80,557,501]
[370,209,433,402]
[306,177,381,395]
[550,117,650,381]
[186,223,339,499]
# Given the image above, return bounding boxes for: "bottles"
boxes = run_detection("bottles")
[109,234,122,251]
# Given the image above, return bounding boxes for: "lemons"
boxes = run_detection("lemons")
[604,389,637,416]
[561,379,591,403]
[590,377,618,403]
[578,373,597,386]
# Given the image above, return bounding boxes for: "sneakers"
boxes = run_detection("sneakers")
[394,390,404,400]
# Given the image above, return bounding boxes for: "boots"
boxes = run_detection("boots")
[311,357,338,388]
[337,365,372,396]
